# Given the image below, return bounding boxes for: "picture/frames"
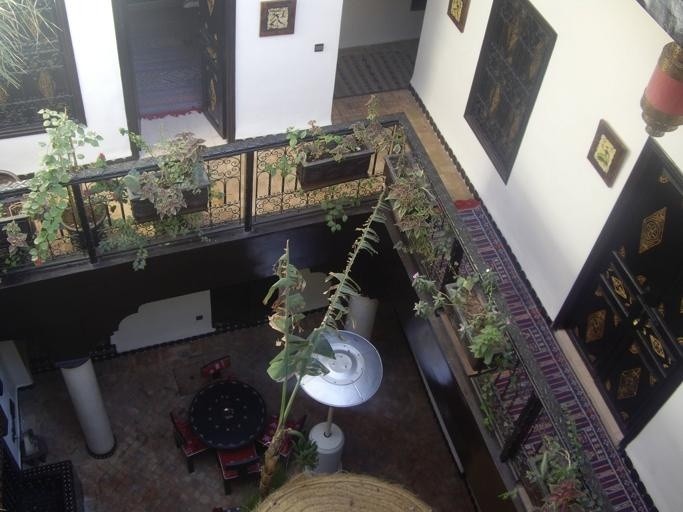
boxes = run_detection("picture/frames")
[586,119,628,188]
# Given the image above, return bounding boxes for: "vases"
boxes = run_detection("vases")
[59,200,107,250]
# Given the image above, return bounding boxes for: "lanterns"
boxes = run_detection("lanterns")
[639,41,683,138]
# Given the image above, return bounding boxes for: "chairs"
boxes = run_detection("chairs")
[199,356,236,382]
[217,441,261,495]
[258,415,309,471]
[168,412,209,474]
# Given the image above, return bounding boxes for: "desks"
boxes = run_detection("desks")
[188,380,266,451]
